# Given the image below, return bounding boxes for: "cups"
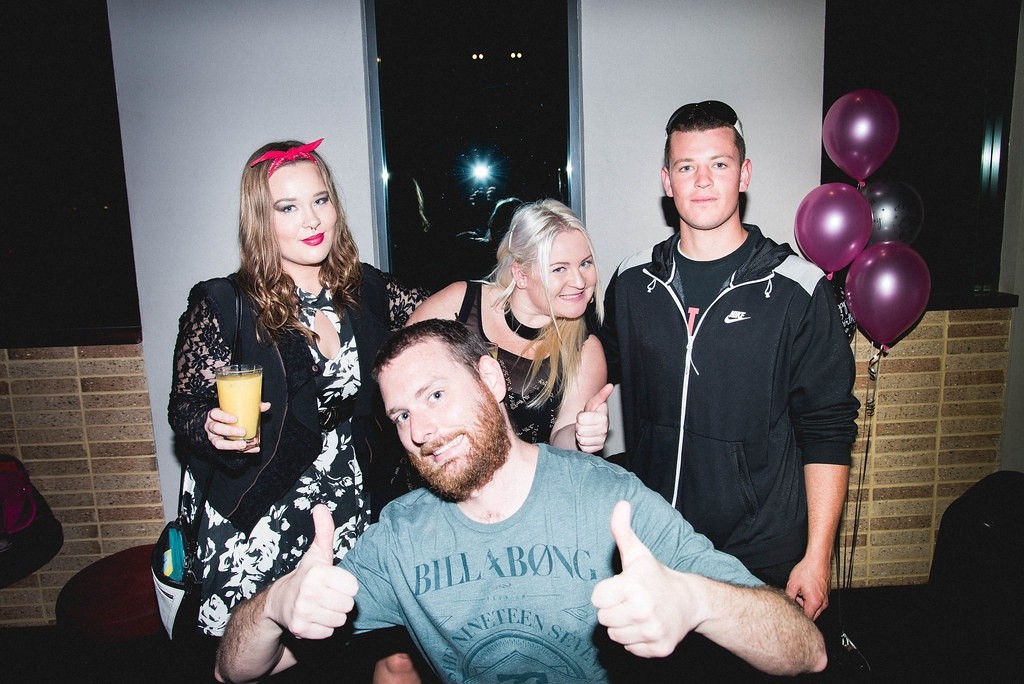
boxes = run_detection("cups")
[215,364,263,446]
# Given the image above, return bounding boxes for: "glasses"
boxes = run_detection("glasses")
[666,100,743,136]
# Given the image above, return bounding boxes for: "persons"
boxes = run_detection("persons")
[214,316,828,684]
[167,140,434,684]
[601,99,860,684]
[368,198,615,684]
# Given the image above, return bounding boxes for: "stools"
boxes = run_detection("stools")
[56,545,161,644]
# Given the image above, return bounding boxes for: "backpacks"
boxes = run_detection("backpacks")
[929,470,1024,635]
[0,454,64,591]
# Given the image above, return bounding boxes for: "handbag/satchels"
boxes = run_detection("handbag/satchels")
[151,514,202,641]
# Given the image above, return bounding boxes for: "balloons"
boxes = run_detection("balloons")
[794,182,872,280]
[844,240,932,352]
[822,88,900,187]
[865,180,925,246]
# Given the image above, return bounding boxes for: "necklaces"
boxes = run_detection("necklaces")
[501,298,543,340]
